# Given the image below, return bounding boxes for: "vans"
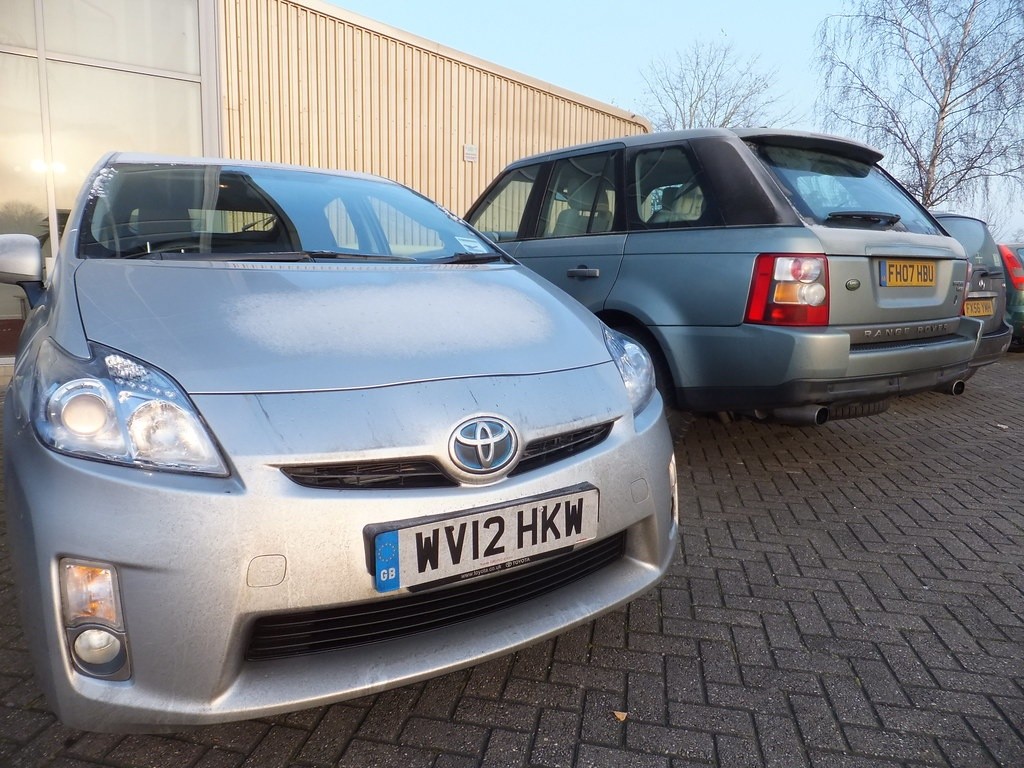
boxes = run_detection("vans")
[925,211,1012,386]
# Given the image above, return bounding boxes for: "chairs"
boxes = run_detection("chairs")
[268,205,332,259]
[136,194,195,253]
[649,187,679,221]
[551,173,613,236]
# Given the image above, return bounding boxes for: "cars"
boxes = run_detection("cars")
[994,242,1024,353]
[0,150,680,736]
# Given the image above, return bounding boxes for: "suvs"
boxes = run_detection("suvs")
[455,128,984,430]
